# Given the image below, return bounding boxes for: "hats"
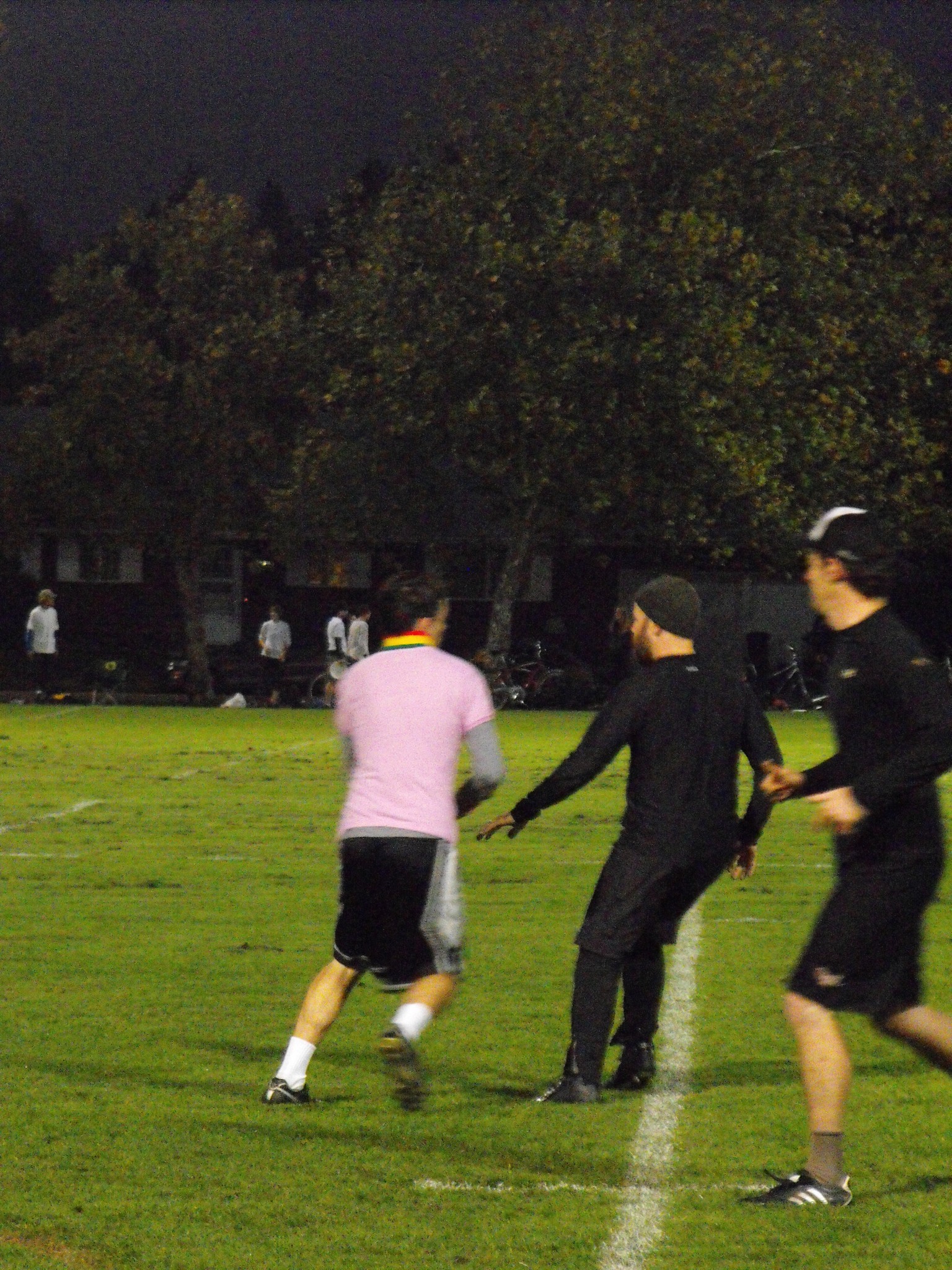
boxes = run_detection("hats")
[783,507,910,577]
[634,577,700,639]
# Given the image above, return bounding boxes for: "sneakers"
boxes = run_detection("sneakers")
[262,1077,312,1106]
[609,1044,657,1089]
[377,1023,424,1113]
[736,1166,855,1210]
[533,1075,605,1102]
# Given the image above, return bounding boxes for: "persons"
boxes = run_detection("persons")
[257,604,292,709]
[26,589,65,705]
[263,569,503,1113]
[476,574,783,1104]
[737,506,952,1207]
[323,604,371,709]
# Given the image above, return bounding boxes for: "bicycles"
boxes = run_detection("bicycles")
[470,637,568,711]
[307,658,351,709]
[746,643,816,710]
